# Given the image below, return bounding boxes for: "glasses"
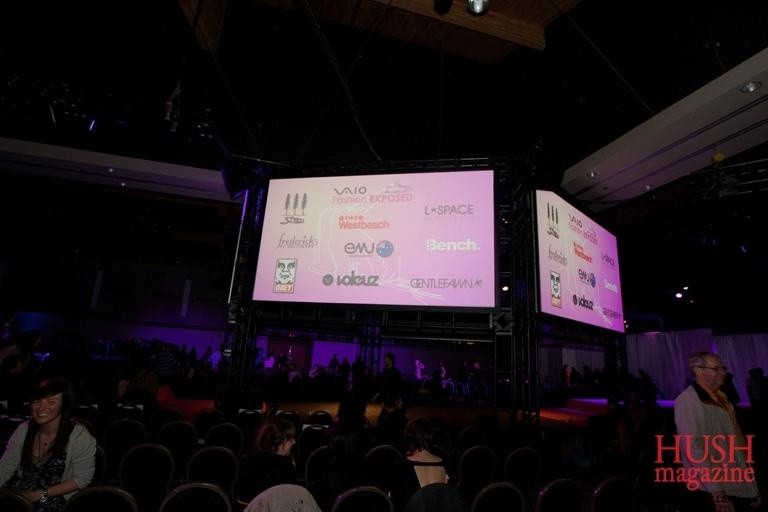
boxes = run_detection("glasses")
[691,364,728,372]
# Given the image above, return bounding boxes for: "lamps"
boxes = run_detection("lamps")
[466,1,490,15]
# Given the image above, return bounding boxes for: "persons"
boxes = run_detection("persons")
[675,350,761,512]
[0,344,657,512]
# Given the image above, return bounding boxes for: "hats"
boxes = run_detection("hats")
[31,379,63,399]
